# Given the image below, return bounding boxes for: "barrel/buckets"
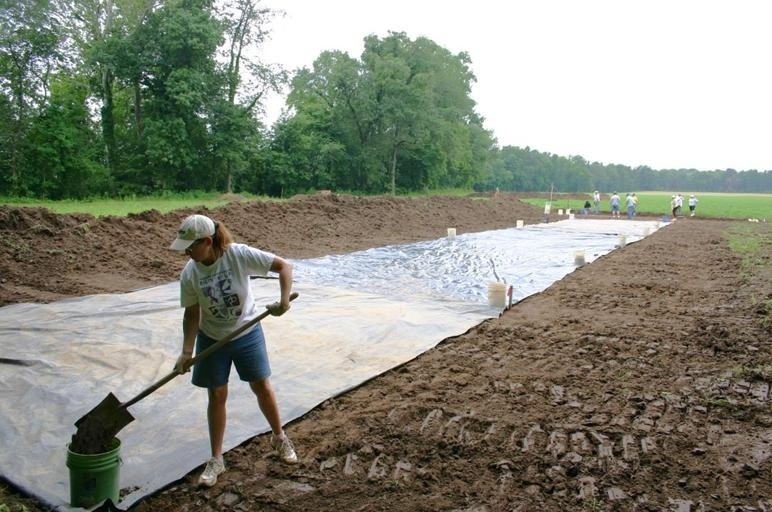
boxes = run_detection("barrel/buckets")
[65,437,122,512]
[516,220,524,229]
[487,283,506,308]
[616,236,625,246]
[568,213,573,219]
[643,224,649,234]
[576,250,585,264]
[447,228,457,243]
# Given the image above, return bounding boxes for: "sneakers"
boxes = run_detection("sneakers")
[270,431,297,465]
[197,456,225,488]
[613,216,621,219]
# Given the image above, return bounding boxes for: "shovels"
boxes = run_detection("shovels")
[75,293,300,448]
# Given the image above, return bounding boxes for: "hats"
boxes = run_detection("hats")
[169,215,216,250]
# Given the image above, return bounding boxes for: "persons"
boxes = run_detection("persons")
[630,193,638,210]
[675,193,684,209]
[624,193,634,219]
[609,191,621,218]
[687,194,696,216]
[168,212,300,489]
[583,201,592,215]
[592,190,601,215]
[182,351,193,357]
[670,195,679,217]
